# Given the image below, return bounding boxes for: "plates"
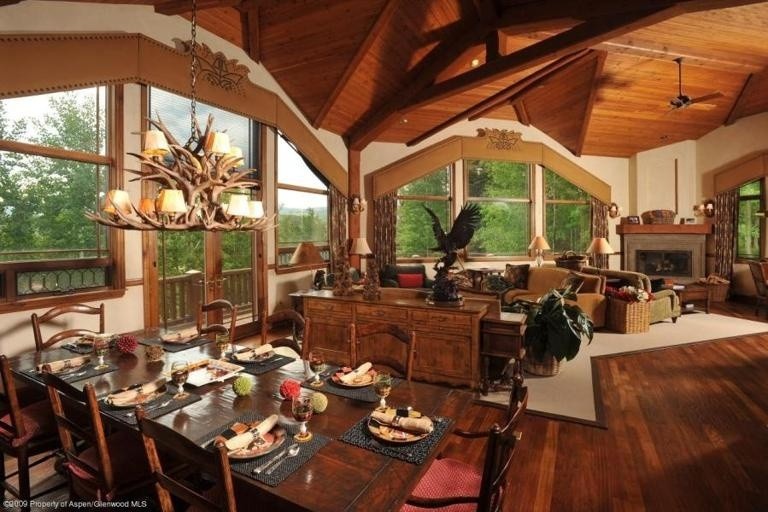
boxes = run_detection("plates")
[231,349,275,363]
[103,385,167,407]
[330,368,376,387]
[212,421,287,460]
[367,409,434,443]
[173,358,245,387]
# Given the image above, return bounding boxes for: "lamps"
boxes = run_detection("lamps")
[289,241,329,292]
[344,234,373,275]
[79,0,314,236]
[586,238,613,269]
[527,234,551,267]
[350,193,369,217]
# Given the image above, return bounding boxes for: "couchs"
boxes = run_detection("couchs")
[479,264,607,331]
[581,265,681,326]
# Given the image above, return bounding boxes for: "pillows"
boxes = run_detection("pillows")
[393,272,426,287]
[503,264,529,289]
[555,270,587,295]
[650,277,667,293]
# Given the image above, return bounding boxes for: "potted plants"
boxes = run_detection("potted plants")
[507,272,595,376]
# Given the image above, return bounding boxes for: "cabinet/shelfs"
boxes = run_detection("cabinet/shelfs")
[288,284,490,400]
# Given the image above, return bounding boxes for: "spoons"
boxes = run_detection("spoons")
[144,400,169,414]
[264,447,300,476]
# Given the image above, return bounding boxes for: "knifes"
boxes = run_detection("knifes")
[252,443,298,474]
[124,403,159,417]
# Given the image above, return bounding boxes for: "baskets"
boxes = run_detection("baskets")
[607,295,650,333]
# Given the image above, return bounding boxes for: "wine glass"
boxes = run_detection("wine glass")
[290,393,314,442]
[214,333,229,361]
[170,361,190,400]
[308,350,326,387]
[93,337,109,371]
[374,371,392,408]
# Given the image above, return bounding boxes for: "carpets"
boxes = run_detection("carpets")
[469,310,768,430]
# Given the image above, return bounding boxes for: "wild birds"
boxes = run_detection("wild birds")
[422,202,482,260]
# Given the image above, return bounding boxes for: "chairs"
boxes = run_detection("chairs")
[747,258,767,317]
[382,261,433,288]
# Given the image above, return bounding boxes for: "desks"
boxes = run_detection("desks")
[477,308,530,395]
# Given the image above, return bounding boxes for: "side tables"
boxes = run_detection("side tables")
[467,268,497,289]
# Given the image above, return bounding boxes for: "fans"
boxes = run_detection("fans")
[666,55,726,115]
